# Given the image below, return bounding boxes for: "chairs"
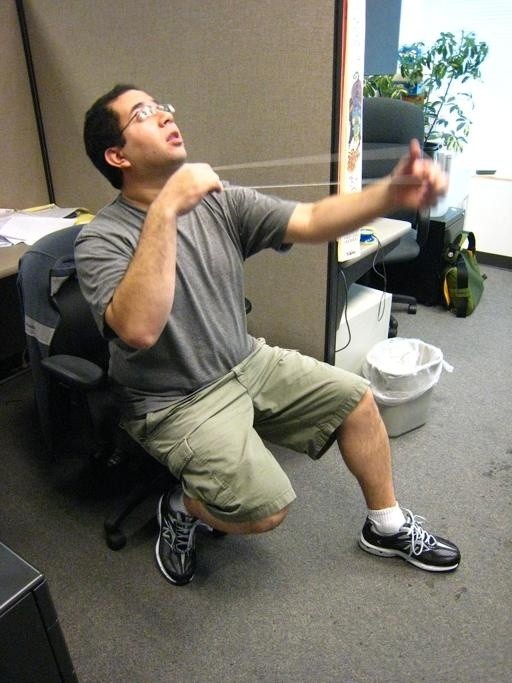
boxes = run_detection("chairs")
[15,221,175,549]
[362,95,428,339]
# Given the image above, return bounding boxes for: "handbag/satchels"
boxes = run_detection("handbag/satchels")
[442,230,487,317]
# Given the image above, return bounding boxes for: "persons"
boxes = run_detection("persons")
[74,86,461,585]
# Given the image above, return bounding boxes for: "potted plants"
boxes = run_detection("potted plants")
[365,28,488,159]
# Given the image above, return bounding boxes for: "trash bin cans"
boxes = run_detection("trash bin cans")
[365,337,444,438]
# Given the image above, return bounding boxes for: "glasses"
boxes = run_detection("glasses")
[118,102,176,140]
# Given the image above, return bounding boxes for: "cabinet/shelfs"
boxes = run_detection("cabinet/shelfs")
[368,207,466,303]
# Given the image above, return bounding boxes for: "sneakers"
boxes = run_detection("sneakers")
[360,513,460,572]
[155,489,197,586]
